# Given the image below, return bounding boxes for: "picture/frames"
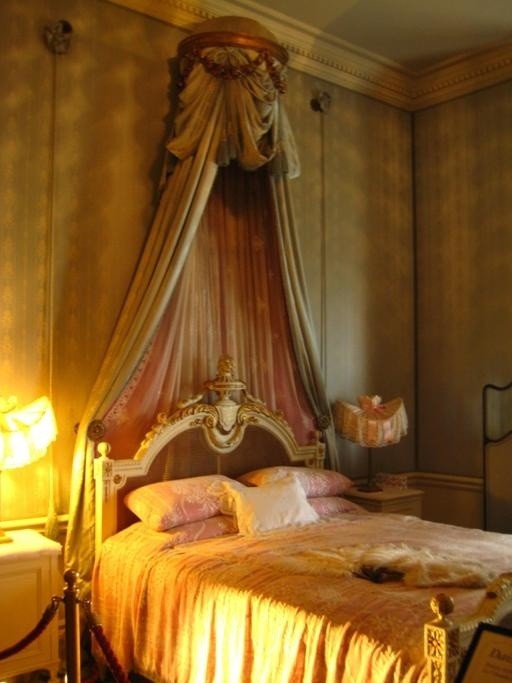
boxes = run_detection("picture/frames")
[452,621,512,683]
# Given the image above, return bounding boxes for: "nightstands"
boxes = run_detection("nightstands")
[0,529,65,683]
[346,482,424,516]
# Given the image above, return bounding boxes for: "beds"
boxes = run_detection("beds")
[92,352,512,683]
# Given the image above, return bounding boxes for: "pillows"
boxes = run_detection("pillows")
[123,474,251,531]
[128,514,238,548]
[235,465,355,499]
[306,496,357,517]
[225,472,319,537]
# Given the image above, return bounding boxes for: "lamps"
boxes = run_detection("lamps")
[0,392,59,543]
[333,394,408,492]
